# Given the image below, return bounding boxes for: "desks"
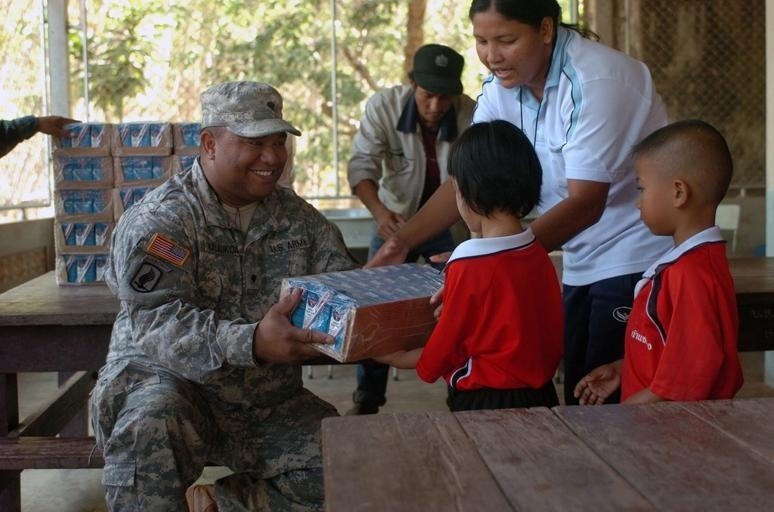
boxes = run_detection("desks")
[727,256,772,352]
[1,272,229,511]
[319,395,774,511]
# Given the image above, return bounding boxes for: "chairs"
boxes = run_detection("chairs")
[715,204,741,256]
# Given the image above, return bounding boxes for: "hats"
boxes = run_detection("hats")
[412,45,463,94]
[200,80,302,138]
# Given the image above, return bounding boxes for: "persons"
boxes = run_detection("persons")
[345,43,478,416]
[0,115,83,159]
[370,118,566,410]
[90,79,361,511]
[573,117,745,407]
[364,0,673,403]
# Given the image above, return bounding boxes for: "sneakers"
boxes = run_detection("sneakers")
[185,483,219,512]
[346,403,379,417]
